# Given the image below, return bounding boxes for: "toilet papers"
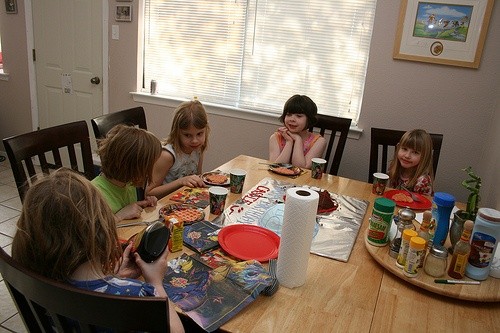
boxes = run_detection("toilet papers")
[275,187,320,289]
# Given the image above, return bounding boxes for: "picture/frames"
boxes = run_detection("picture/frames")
[115,5,131,21]
[4,0,17,14]
[392,0,495,69]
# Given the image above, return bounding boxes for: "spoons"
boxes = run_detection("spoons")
[401,186,422,202]
[116,218,164,228]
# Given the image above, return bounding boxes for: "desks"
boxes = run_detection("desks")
[110,155,500,333]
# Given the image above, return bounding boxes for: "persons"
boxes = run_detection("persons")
[10,166,185,333]
[91,122,162,224]
[269,94,326,169]
[144,99,209,200]
[387,129,434,196]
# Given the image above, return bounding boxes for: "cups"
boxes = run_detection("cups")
[208,187,228,215]
[230,170,247,194]
[372,172,389,195]
[310,157,327,179]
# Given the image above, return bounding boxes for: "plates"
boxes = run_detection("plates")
[200,170,231,185]
[159,202,205,224]
[269,162,302,175]
[218,224,280,263]
[283,195,338,213]
[382,189,432,210]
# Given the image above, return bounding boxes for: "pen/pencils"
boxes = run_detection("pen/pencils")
[435,280,480,284]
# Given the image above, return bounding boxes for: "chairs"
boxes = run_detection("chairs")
[0,105,442,333]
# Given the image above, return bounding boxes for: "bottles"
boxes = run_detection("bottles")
[366,191,500,280]
